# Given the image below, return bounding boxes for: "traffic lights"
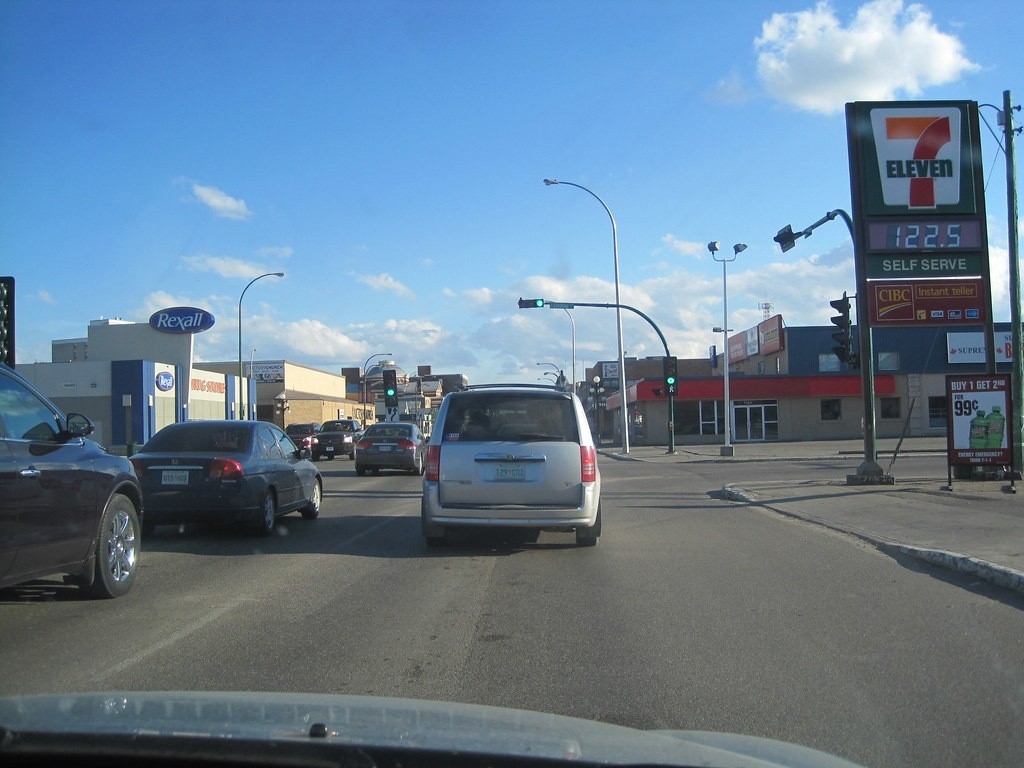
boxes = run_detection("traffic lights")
[773,224,795,252]
[383,370,398,407]
[663,356,678,397]
[829,291,853,361]
[518,297,544,309]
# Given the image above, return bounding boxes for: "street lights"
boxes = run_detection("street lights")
[590,376,604,448]
[544,300,576,394]
[538,378,556,385]
[544,372,558,378]
[276,394,290,430]
[542,179,629,454]
[366,364,383,375]
[250,348,257,378]
[364,353,392,429]
[537,363,559,373]
[707,240,748,456]
[239,273,285,420]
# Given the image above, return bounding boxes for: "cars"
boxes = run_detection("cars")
[0,361,144,598]
[311,420,364,461]
[129,420,323,535]
[355,423,430,476]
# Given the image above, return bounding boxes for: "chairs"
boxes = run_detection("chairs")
[375,429,408,437]
[462,421,490,437]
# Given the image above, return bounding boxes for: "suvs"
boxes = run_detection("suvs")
[285,422,321,454]
[421,387,601,546]
